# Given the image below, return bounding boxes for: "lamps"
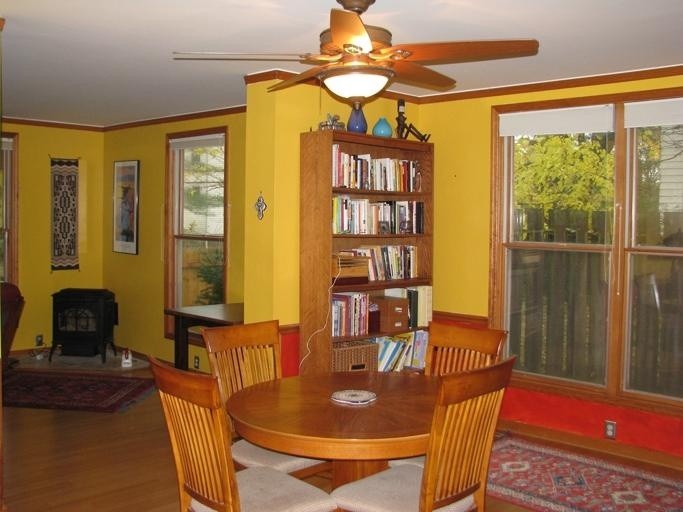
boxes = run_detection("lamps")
[314,55,396,112]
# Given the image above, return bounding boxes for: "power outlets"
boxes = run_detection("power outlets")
[194,356,200,369]
[36,336,43,346]
[605,419,616,441]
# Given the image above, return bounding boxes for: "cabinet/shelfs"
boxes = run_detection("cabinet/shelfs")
[300,128,434,375]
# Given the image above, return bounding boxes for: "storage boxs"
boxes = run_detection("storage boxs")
[370,296,409,333]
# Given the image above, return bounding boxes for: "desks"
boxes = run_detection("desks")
[163,302,252,372]
[225,370,441,512]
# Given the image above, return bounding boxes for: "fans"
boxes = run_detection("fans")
[173,0,539,91]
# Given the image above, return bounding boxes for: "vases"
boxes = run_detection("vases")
[372,116,392,137]
[346,105,367,134]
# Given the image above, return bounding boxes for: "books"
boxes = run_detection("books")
[332,141,429,373]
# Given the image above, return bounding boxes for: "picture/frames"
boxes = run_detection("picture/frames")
[112,159,140,255]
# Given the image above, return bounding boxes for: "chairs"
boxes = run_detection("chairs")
[388,321,509,469]
[1,280,26,380]
[330,354,518,512]
[199,319,333,492]
[147,353,337,512]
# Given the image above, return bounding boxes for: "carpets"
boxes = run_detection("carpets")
[484,432,683,512]
[2,368,158,414]
